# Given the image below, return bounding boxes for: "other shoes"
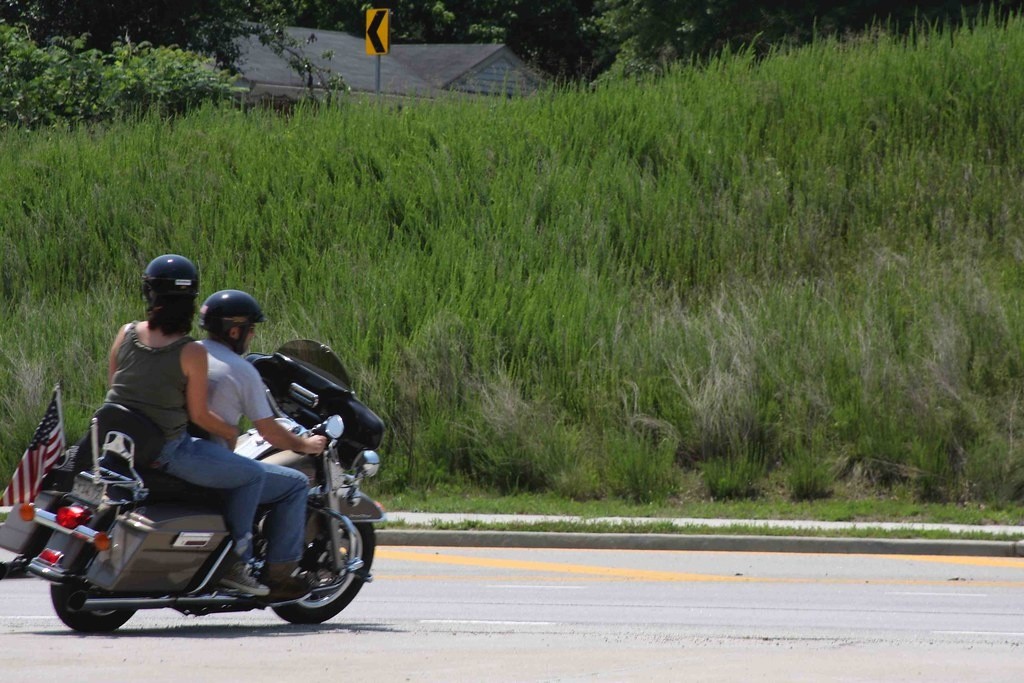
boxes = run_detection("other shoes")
[221,569,271,596]
[256,562,320,600]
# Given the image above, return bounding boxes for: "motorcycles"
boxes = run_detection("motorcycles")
[0,339,388,632]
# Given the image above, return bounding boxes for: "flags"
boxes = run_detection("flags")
[0,384,66,507]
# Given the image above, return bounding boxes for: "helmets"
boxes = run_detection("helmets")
[198,290,267,335]
[142,253,198,295]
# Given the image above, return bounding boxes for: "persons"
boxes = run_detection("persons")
[194,289,327,603]
[103,254,271,597]
